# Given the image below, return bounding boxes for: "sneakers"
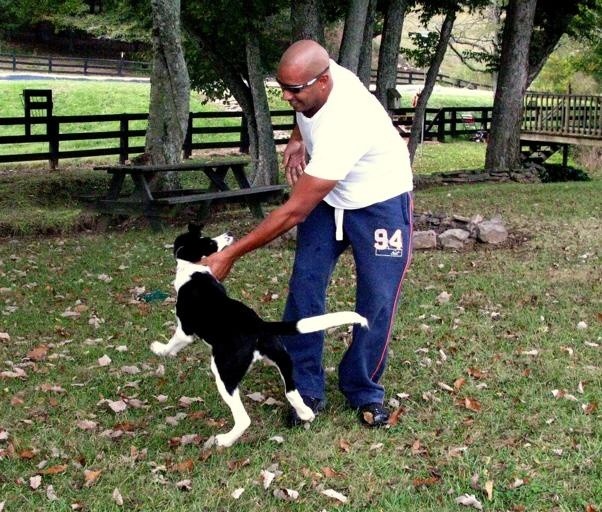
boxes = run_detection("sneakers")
[349,398,389,429]
[286,393,328,429]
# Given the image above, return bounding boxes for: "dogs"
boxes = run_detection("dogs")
[150,221,371,449]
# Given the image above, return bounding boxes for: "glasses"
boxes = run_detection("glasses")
[275,65,330,93]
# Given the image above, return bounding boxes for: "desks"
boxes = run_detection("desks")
[520,133,576,167]
[93,157,264,234]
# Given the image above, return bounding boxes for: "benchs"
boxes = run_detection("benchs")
[72,184,290,211]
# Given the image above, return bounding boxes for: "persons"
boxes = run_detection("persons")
[195,38,415,429]
